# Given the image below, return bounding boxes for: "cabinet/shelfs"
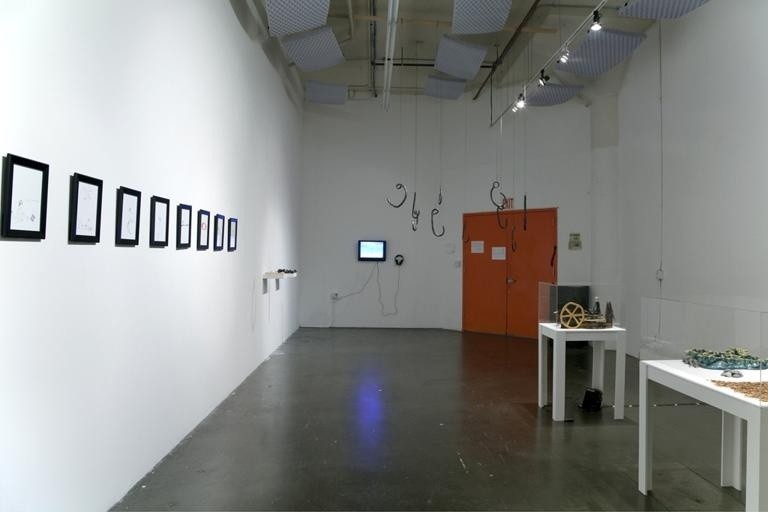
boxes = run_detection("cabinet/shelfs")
[538,281,589,346]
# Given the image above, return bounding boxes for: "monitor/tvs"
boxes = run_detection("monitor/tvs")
[358,240,386,262]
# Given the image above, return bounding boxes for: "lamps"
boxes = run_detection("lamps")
[381,0,399,112]
[489,0,607,126]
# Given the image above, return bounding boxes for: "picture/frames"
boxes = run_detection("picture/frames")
[178,204,192,247]
[214,214,225,250]
[150,196,169,246]
[229,218,237,250]
[116,186,141,245]
[198,209,210,249]
[71,173,103,242]
[2,153,49,239]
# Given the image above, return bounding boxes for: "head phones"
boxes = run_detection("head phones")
[395,255,404,265]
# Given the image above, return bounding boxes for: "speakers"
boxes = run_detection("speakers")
[565,399,577,422]
[582,388,602,412]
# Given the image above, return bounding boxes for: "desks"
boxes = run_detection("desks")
[638,359,768,512]
[538,323,626,422]
